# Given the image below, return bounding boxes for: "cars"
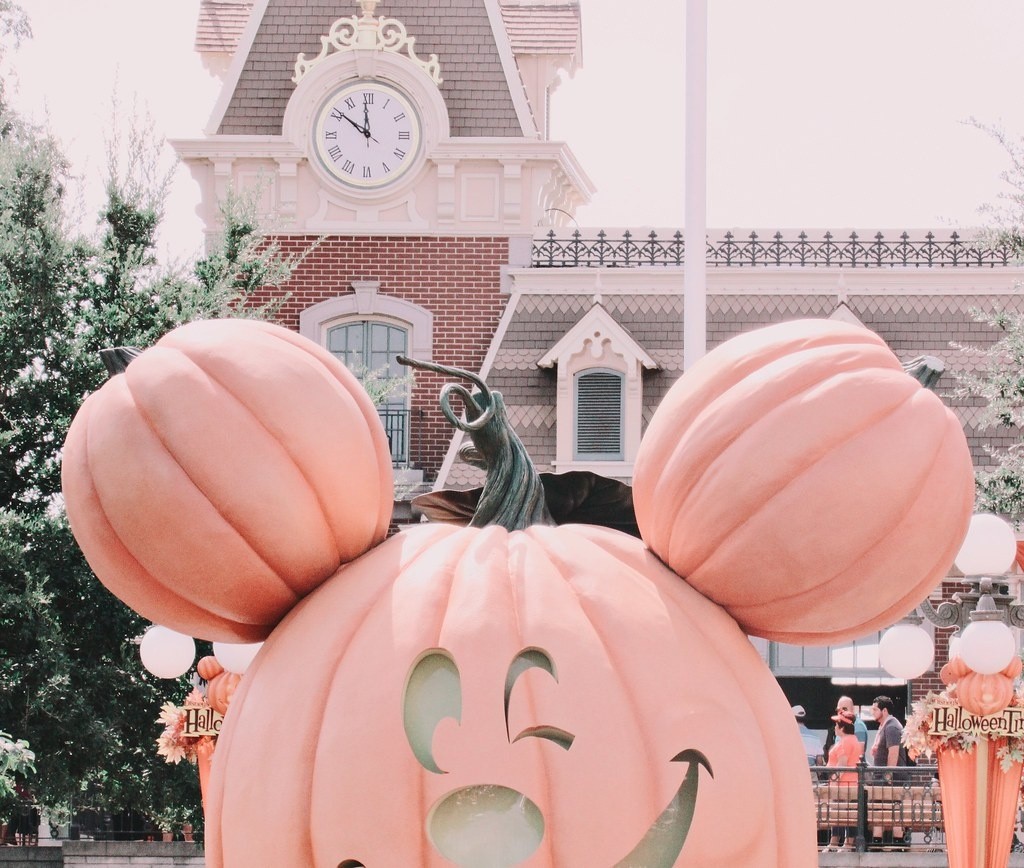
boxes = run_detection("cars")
[69,805,164,841]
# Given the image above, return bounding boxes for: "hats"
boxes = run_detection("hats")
[792,705,806,717]
[831,711,856,726]
[836,696,854,711]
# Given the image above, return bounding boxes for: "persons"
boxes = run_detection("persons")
[866,695,907,852]
[791,696,868,852]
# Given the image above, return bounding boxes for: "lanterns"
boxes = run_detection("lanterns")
[959,671,1013,716]
[206,671,242,715]
[204,526,818,867]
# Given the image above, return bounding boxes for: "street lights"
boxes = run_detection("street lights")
[880,514,1024,868]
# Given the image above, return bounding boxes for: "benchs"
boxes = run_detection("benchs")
[812,783,944,833]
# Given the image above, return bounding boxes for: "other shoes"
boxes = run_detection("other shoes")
[822,847,838,852]
[838,846,852,852]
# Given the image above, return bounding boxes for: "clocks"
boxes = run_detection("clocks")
[307,72,430,201]
